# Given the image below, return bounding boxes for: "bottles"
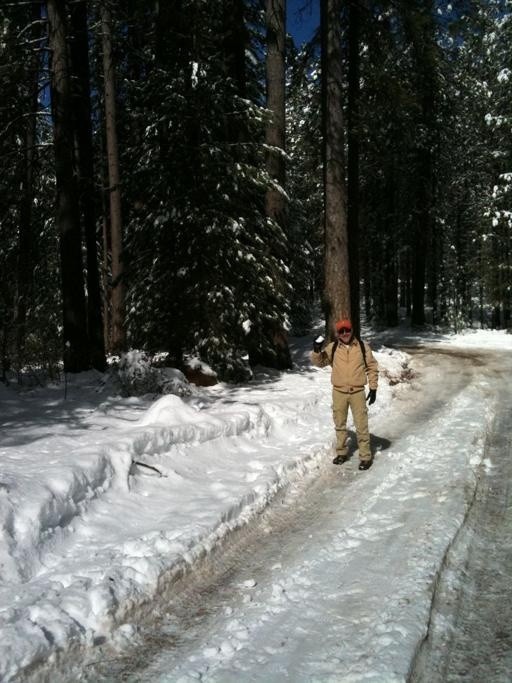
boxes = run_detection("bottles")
[335,318,352,330]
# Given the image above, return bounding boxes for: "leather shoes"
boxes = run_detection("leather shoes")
[313,334,325,354]
[365,388,377,406]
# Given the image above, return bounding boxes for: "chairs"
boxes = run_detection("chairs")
[333,454,346,464]
[358,459,374,471]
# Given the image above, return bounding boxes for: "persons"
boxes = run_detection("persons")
[310,319,379,470]
